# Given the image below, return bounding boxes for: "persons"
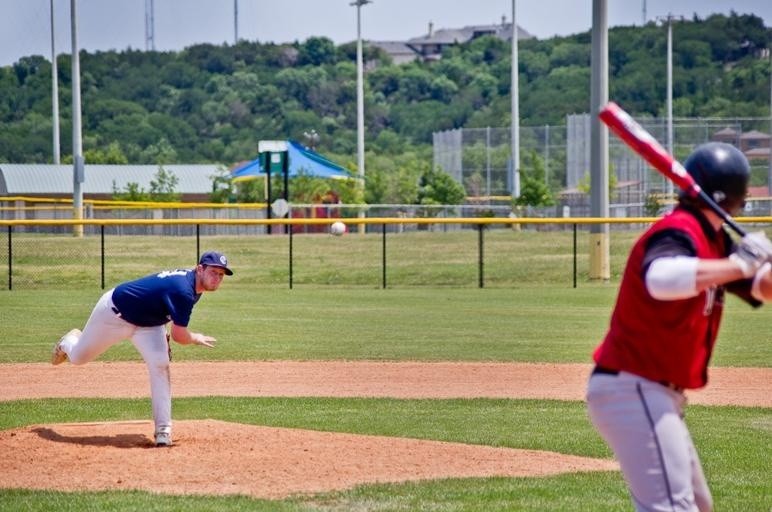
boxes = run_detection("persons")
[50,250,234,448]
[270,188,349,234]
[585,141,772,512]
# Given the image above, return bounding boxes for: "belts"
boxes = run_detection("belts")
[592,366,689,395]
[112,307,126,320]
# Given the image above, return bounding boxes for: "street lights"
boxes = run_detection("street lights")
[349,0,374,233]
[655,11,684,214]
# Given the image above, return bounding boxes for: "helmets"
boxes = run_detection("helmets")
[677,141,751,209]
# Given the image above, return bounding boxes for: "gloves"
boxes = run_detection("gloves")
[727,232,771,277]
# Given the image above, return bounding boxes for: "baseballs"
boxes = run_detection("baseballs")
[330,222,347,235]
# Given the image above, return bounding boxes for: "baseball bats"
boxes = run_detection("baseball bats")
[598,100,747,240]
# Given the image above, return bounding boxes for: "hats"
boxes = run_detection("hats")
[199,250,234,276]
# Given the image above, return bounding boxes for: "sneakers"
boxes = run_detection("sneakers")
[154,432,172,447]
[51,328,82,365]
